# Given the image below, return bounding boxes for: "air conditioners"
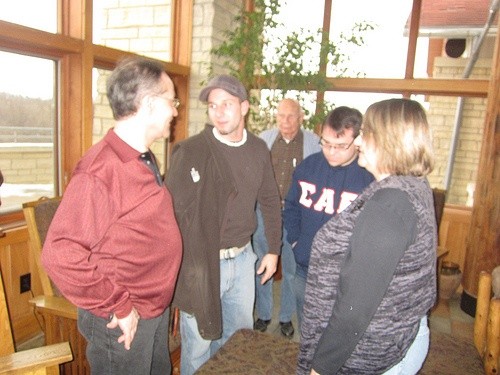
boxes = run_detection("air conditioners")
[442,37,471,58]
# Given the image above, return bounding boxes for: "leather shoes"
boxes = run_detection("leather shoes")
[253,318,271,333]
[279,321,296,339]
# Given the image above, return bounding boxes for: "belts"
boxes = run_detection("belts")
[218,241,251,259]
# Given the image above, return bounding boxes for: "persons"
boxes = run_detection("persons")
[41,57,182,375]
[253,98,325,338]
[279,106,378,334]
[163,73,284,375]
[296,98,437,375]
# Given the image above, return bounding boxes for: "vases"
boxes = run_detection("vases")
[439,262,462,299]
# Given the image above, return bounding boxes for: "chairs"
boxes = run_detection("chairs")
[416,271,500,374]
[432,188,449,262]
[22,197,181,375]
[194,328,299,374]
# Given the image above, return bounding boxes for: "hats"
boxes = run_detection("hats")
[199,73,247,104]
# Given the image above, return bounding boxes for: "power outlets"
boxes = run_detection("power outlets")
[20,273,31,294]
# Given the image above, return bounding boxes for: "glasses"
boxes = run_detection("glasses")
[318,136,357,152]
[155,94,180,108]
